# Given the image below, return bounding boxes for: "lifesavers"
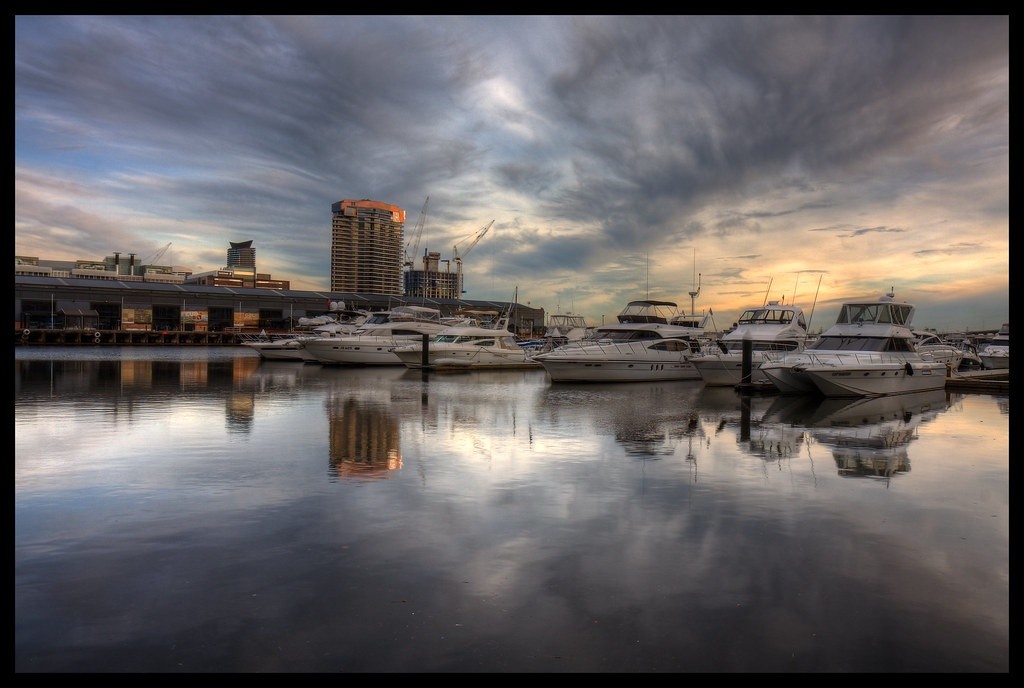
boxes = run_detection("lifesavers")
[23,329,30,335]
[22,335,29,340]
[95,332,100,337]
[95,338,100,343]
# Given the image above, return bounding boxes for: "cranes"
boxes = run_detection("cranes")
[402,196,431,266]
[144,242,173,264]
[450,219,500,298]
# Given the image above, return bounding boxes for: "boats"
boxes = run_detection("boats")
[682,303,821,387]
[533,299,712,383]
[238,315,326,360]
[392,318,537,370]
[297,306,457,363]
[978,321,1008,370]
[759,300,964,396]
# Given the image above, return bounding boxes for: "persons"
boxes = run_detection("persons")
[328,298,332,310]
